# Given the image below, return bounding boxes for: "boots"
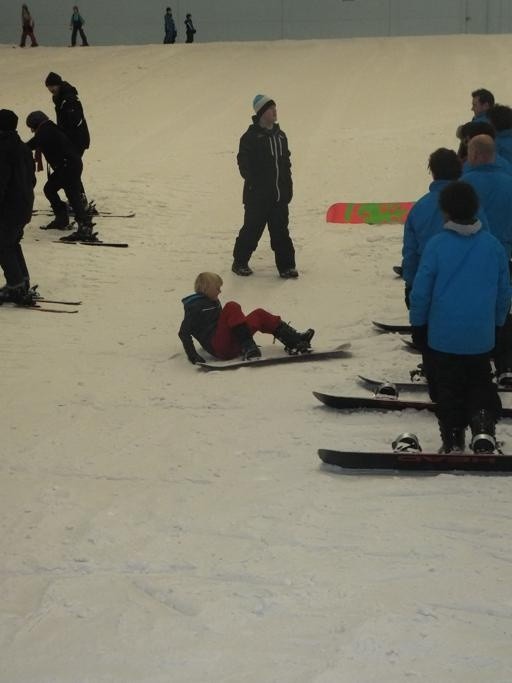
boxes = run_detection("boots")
[48,192,92,240]
[437,429,465,454]
[274,321,315,353]
[471,408,495,451]
[233,324,261,358]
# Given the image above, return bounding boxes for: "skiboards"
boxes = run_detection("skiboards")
[13,298,81,314]
[40,224,128,247]
[34,208,136,217]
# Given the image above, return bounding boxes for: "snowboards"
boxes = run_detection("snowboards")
[319,448,511,470]
[359,375,427,391]
[373,321,414,334]
[314,392,512,415]
[326,203,415,224]
[196,344,349,370]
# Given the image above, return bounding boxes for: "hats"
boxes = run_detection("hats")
[0,109,18,130]
[46,72,62,86]
[253,95,275,120]
[26,111,49,130]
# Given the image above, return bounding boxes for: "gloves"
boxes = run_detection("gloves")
[410,325,429,353]
[188,354,205,366]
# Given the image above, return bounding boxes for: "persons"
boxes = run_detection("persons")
[184,14,196,43]
[164,7,175,44]
[70,7,88,47]
[403,90,512,455]
[24,111,93,238]
[45,72,90,212]
[0,110,36,302]
[178,272,314,364]
[232,95,298,278]
[20,4,38,46]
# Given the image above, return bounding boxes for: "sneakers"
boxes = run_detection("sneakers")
[393,266,403,276]
[280,268,299,277]
[231,261,252,277]
[496,366,512,385]
[0,280,30,299]
